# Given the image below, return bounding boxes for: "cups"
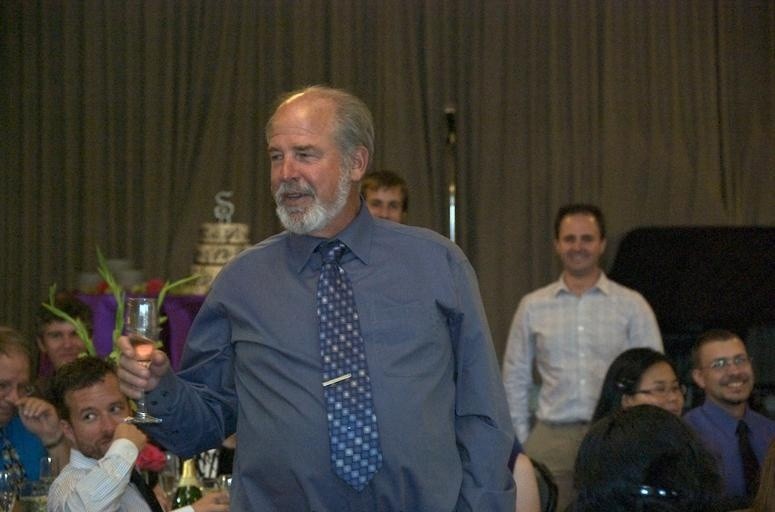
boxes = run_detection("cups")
[158,452,181,508]
[39,456,58,482]
[214,473,231,505]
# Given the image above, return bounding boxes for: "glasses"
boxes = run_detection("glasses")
[631,384,688,397]
[696,356,752,373]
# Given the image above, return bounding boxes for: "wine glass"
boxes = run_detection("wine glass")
[119,298,163,425]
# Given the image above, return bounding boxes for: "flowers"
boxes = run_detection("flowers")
[41,243,204,424]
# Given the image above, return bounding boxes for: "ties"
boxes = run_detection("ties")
[319,242,383,494]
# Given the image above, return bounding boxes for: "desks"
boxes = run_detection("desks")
[35,291,221,387]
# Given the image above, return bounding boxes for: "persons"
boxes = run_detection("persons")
[357,169,411,228]
[0,294,774,512]
[116,85,518,512]
[500,200,666,510]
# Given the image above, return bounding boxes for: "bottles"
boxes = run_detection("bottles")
[173,458,203,510]
